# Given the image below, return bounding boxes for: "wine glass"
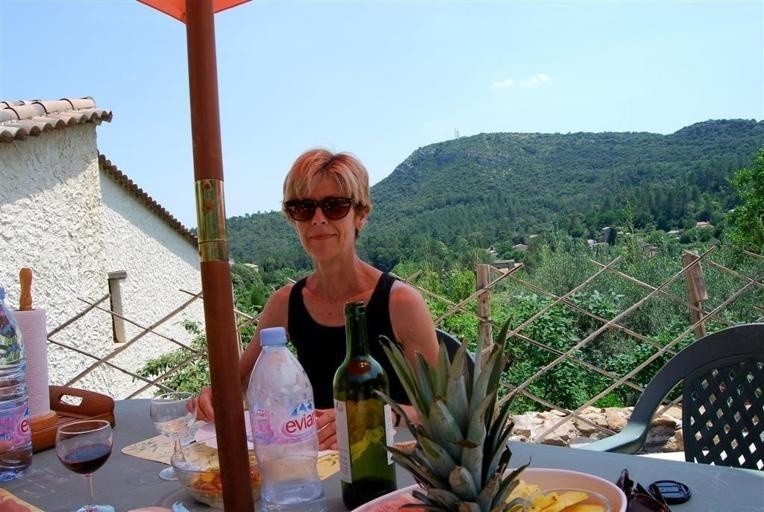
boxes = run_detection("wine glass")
[53,392,198,512]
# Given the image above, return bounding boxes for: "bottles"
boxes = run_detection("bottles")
[248,301,399,512]
[0,287,32,483]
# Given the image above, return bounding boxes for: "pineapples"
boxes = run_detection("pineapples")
[373,314,606,512]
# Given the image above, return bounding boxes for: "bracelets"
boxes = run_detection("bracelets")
[391,406,402,427]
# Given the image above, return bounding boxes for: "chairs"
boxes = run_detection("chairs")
[575,322,764,471]
[433,326,476,382]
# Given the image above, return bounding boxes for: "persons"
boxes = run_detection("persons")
[189,149,440,452]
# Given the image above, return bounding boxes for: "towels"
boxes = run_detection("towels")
[121,418,359,487]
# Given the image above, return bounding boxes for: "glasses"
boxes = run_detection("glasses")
[283,196,356,222]
[617,469,673,511]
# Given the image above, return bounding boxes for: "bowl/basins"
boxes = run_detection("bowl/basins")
[171,434,264,507]
[347,468,628,512]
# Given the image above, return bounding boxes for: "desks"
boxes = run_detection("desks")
[0,399,764,512]
